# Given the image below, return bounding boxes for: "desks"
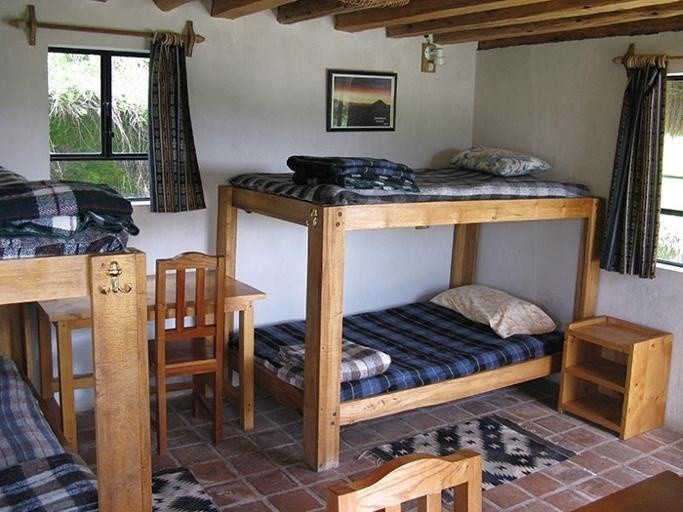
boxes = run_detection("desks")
[33,268,267,456]
[570,470,682,512]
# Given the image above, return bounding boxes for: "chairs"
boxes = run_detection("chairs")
[148,250,227,456]
[324,450,484,512]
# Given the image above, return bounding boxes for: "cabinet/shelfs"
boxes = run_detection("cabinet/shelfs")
[554,315,672,441]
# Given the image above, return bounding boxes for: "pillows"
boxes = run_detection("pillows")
[446,143,552,179]
[428,284,558,341]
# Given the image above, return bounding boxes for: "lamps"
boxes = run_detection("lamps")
[421,33,445,74]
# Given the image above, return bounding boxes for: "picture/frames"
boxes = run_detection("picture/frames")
[325,69,396,132]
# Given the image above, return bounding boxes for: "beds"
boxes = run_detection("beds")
[0,171,155,512]
[216,168,605,475]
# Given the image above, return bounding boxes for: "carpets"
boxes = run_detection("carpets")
[356,414,582,506]
[148,465,227,512]
